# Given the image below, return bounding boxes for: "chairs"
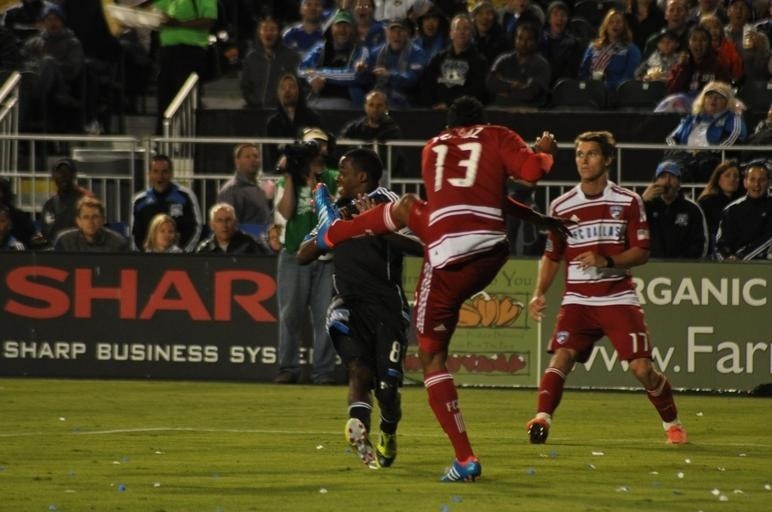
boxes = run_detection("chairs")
[32,219,42,233]
[555,81,607,108]
[236,223,269,239]
[322,9,336,20]
[103,222,129,236]
[571,18,591,33]
[613,78,669,111]
[740,80,772,115]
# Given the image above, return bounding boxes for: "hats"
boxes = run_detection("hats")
[51,157,78,172]
[387,16,410,31]
[655,160,683,178]
[703,81,730,101]
[302,128,328,144]
[42,4,65,20]
[655,28,679,40]
[334,10,352,24]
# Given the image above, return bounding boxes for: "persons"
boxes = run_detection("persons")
[275,129,339,250]
[297,9,370,102]
[144,214,186,254]
[644,0,697,61]
[421,13,490,108]
[4,1,71,50]
[215,144,273,240]
[39,158,94,242]
[262,73,325,169]
[543,1,582,79]
[580,10,642,105]
[413,7,451,59]
[298,148,425,467]
[260,223,285,257]
[634,31,680,83]
[698,16,743,87]
[338,91,409,194]
[53,196,128,255]
[197,204,272,258]
[641,161,708,260]
[350,1,388,47]
[311,95,559,482]
[678,27,721,97]
[490,21,550,107]
[239,21,300,105]
[528,133,687,446]
[283,1,325,59]
[153,1,217,153]
[356,17,427,109]
[36,5,82,86]
[716,160,771,263]
[722,1,769,81]
[470,4,506,67]
[691,1,728,28]
[1,205,25,254]
[665,82,747,197]
[697,161,742,259]
[130,155,200,249]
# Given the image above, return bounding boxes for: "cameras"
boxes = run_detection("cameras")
[275,140,320,186]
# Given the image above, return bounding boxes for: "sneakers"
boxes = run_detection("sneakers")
[312,373,336,385]
[276,369,302,383]
[440,456,481,482]
[344,417,375,464]
[527,418,549,445]
[376,431,398,467]
[310,182,338,250]
[664,421,689,444]
